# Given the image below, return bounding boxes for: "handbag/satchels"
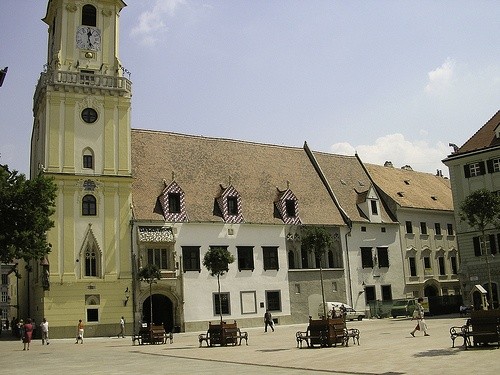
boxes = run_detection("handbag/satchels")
[419,321,428,330]
[416,323,420,330]
[78,335,81,340]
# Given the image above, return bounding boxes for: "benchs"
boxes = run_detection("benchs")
[199,320,249,347]
[450,309,500,350]
[132,323,174,345]
[297,313,361,348]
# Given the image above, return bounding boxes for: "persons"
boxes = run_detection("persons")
[6,318,9,330]
[117,317,125,338]
[22,318,33,350]
[39,318,49,345]
[331,307,336,318]
[10,317,37,340]
[410,297,430,337]
[76,320,84,344]
[339,305,347,323]
[264,309,274,332]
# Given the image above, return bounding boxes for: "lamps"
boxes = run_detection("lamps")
[359,280,367,295]
[122,286,132,306]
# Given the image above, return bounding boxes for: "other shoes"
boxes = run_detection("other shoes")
[424,334,430,336]
[410,332,415,337]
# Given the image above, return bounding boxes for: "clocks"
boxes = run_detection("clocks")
[76,26,101,51]
